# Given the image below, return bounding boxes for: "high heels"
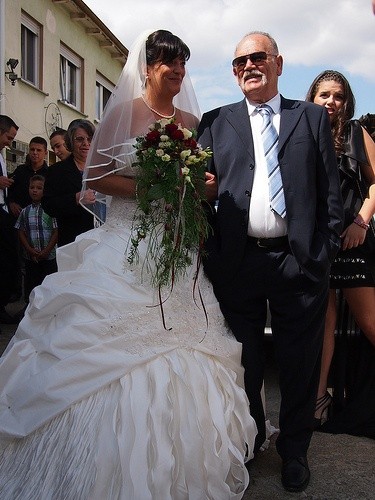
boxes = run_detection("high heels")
[311,389,335,431]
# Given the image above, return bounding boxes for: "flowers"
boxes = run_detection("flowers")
[124,117,215,289]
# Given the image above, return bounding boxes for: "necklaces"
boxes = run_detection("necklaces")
[142,94,177,119]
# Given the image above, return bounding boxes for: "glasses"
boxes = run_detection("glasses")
[231,51,275,68]
[71,135,92,144]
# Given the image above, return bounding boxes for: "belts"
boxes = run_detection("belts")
[246,236,289,249]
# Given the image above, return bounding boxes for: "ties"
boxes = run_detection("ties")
[255,104,287,219]
[0,162,8,207]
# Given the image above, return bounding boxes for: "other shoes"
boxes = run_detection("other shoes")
[17,287,22,297]
[8,293,17,302]
[0,310,18,324]
[15,304,28,321]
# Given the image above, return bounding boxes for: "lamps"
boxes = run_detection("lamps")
[4,58,19,86]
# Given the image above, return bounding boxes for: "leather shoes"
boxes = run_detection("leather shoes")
[244,443,258,466]
[281,454,310,493]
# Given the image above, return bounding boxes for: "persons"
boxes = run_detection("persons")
[0,27,255,500]
[0,114,19,326]
[48,125,72,160]
[6,136,49,304]
[304,70,375,428]
[43,118,105,246]
[180,31,345,494]
[13,173,67,305]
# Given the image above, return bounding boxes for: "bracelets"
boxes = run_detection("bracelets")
[353,218,369,231]
